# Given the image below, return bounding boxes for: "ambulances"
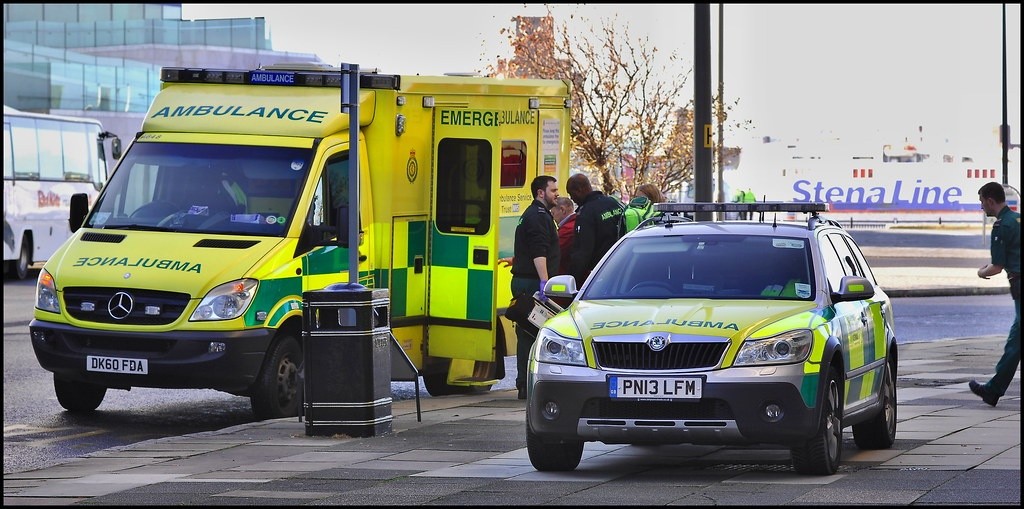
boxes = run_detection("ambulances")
[28,62,573,422]
[526,202,898,476]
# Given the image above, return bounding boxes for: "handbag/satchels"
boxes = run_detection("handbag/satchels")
[505,290,565,339]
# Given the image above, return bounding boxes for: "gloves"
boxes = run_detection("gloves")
[538,280,548,302]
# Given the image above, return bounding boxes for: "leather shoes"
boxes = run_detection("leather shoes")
[518,386,527,399]
[969,380,1000,407]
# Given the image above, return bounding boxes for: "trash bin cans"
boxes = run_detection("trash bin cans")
[301,283,391,437]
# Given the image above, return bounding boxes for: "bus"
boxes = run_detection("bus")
[3,104,122,281]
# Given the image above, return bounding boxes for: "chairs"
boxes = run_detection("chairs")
[659,251,705,292]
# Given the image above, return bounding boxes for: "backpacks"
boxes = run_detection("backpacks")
[609,196,662,239]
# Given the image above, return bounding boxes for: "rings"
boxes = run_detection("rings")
[503,258,504,260]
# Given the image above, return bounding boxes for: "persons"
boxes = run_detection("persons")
[498,173,668,399]
[731,188,757,220]
[969,182,1020,407]
